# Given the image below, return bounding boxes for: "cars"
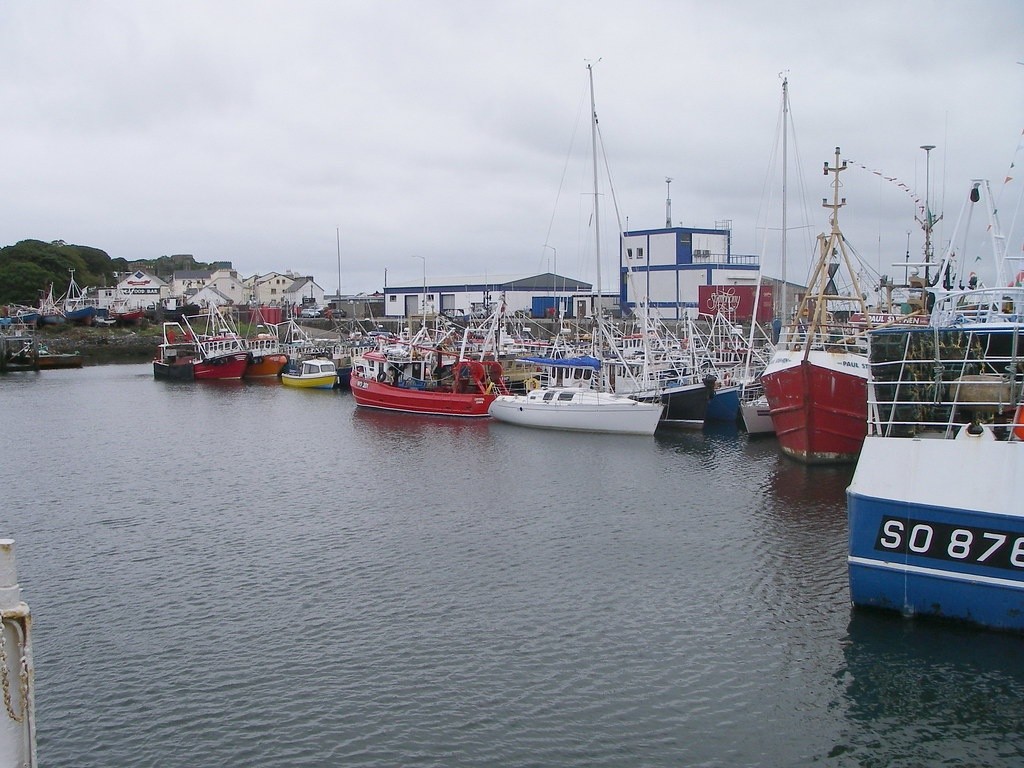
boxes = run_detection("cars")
[329,309,347,318]
[301,309,320,318]
[472,307,487,319]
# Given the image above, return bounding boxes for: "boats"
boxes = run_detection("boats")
[0,268,145,329]
[152,58,1024,636]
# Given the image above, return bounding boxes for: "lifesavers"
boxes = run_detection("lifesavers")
[211,359,217,366]
[202,358,209,366]
[526,377,539,391]
[376,372,387,383]
[218,358,225,365]
[257,356,264,363]
